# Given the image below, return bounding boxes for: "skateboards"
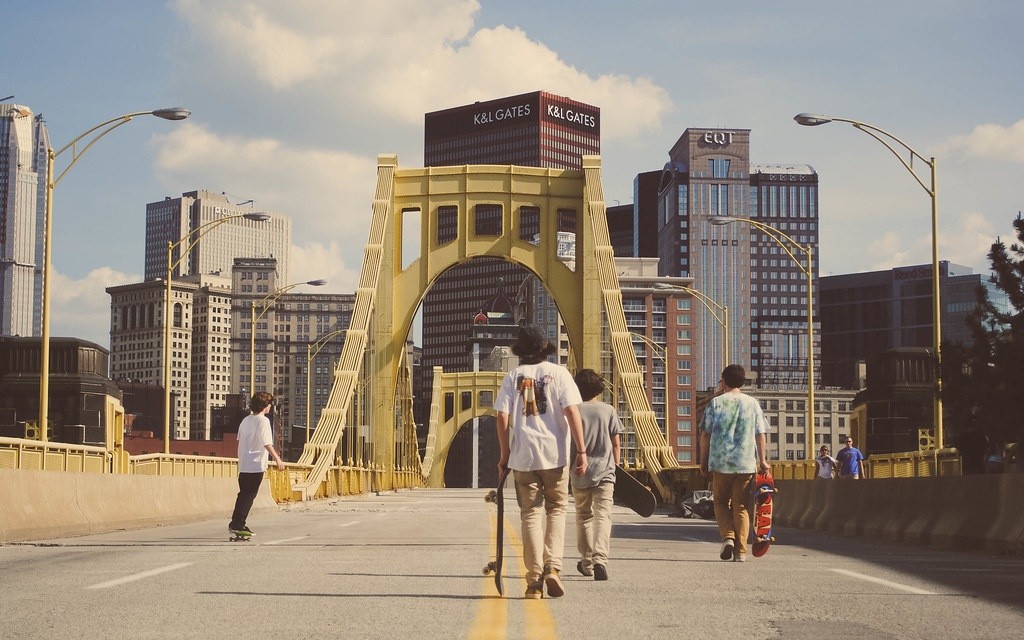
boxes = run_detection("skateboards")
[227,529,252,542]
[752,470,781,557]
[482,468,511,596]
[614,463,656,518]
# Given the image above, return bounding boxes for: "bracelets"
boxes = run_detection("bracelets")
[576,450,586,453]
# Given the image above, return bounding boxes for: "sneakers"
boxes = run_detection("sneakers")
[544,568,565,597]
[525,583,543,600]
[228,522,256,536]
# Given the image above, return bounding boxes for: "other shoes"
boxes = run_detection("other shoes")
[577,562,592,576]
[594,564,608,581]
[720,538,745,562]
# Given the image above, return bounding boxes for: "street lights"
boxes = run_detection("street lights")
[249,279,328,416]
[630,331,669,445]
[793,112,943,450]
[653,282,729,370]
[306,329,347,441]
[38,107,192,441]
[162,212,270,455]
[706,213,815,458]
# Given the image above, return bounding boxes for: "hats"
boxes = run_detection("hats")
[512,325,557,358]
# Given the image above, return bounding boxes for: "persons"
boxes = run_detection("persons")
[833,436,867,479]
[569,369,627,581]
[227,391,286,536]
[491,323,590,601]
[814,445,839,480]
[698,363,771,562]
